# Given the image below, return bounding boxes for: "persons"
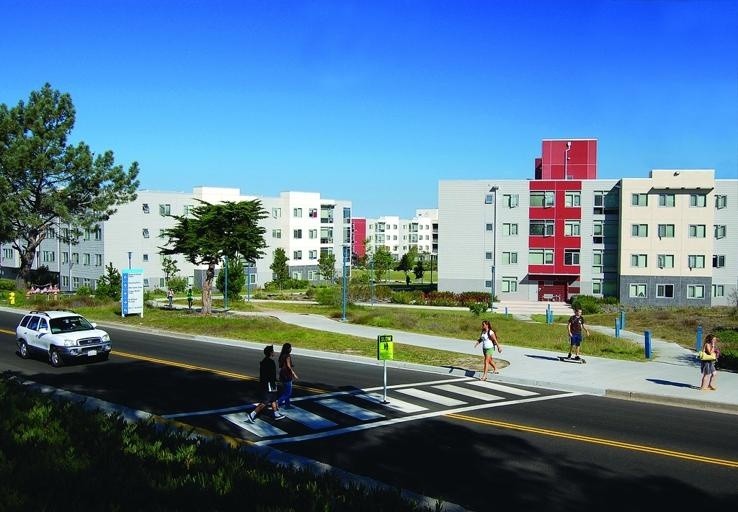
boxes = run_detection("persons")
[698,335,719,392]
[167,288,174,309]
[278,345,299,411]
[474,321,503,381]
[246,344,286,425]
[185,288,194,310]
[567,308,590,360]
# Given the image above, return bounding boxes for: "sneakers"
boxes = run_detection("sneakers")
[248,414,255,424]
[274,414,285,420]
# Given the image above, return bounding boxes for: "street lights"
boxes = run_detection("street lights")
[370,260,374,304]
[340,242,350,320]
[488,186,498,313]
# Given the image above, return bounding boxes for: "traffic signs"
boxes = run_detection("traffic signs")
[122,273,143,281]
[123,307,143,314]
[123,298,142,306]
[123,282,143,290]
[123,290,142,297]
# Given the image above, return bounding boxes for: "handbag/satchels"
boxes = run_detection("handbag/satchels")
[283,370,292,382]
[699,351,717,361]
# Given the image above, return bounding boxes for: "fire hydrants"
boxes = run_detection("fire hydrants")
[8,292,15,305]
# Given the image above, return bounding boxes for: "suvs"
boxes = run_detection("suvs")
[15,308,112,368]
